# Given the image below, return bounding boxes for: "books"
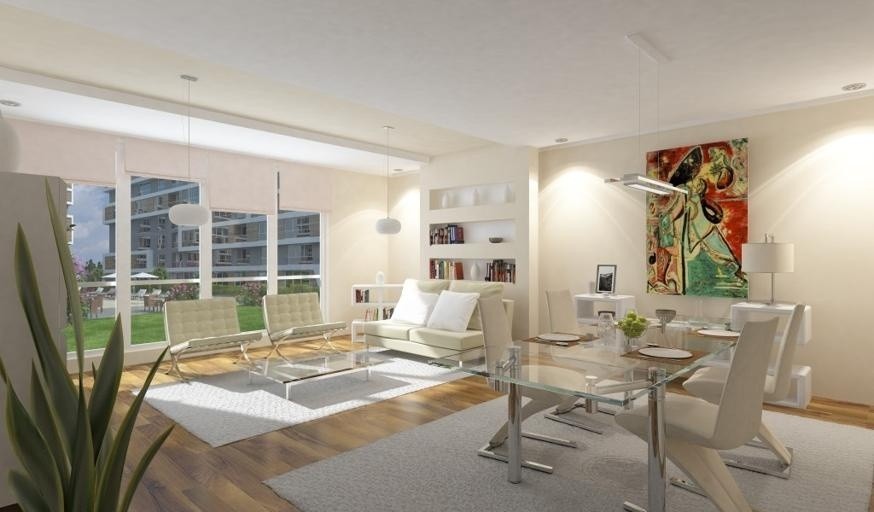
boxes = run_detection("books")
[431,225,465,244]
[486,260,515,282]
[355,288,373,303]
[430,259,464,279]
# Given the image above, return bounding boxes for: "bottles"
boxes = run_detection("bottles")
[598,313,613,344]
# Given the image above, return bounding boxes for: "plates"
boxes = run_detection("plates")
[542,334,580,341]
[698,330,739,337]
[639,347,692,358]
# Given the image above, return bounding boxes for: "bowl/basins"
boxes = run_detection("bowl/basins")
[656,309,676,323]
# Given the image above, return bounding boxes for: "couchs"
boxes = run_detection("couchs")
[364,280,514,368]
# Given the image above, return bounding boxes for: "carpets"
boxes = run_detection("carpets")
[261,376,874,512]
[131,346,468,449]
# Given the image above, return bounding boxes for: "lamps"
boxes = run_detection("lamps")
[169,76,213,227]
[742,233,794,307]
[375,125,402,235]
[620,33,689,197]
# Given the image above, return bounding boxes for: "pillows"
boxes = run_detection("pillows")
[391,293,437,327]
[428,291,479,331]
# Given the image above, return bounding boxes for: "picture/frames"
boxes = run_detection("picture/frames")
[595,264,616,293]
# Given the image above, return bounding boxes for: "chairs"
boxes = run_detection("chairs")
[614,316,781,512]
[544,289,641,435]
[478,295,577,474]
[163,298,262,384]
[683,303,805,481]
[263,292,346,362]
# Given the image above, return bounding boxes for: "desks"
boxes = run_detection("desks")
[639,316,732,352]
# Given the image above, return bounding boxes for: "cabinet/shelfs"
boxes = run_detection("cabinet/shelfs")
[420,144,539,339]
[577,293,636,323]
[351,284,403,343]
[731,301,812,410]
